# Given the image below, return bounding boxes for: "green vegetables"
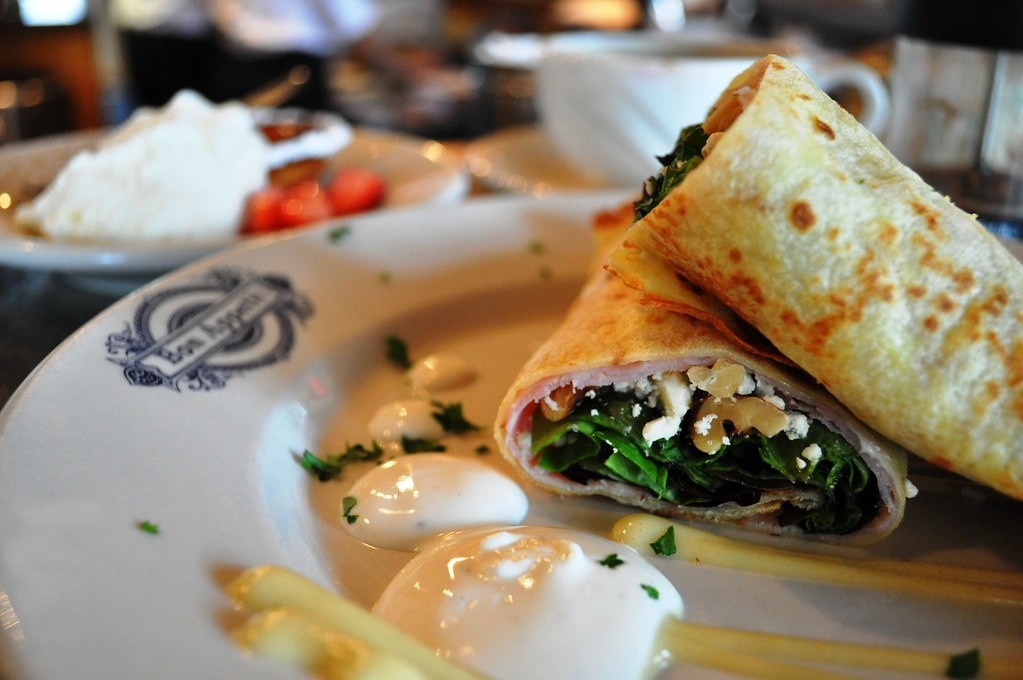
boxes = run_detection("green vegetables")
[137,337,678,597]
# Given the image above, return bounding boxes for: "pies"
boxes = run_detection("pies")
[604,52,1022,501]
[491,192,907,549]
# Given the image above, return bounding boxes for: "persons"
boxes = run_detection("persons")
[111,0,381,111]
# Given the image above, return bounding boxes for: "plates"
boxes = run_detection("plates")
[0,127,472,300]
[0,180,1023,680]
[465,123,624,193]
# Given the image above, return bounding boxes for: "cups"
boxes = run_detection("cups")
[533,28,891,190]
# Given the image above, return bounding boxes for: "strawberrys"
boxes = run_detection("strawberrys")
[247,126,387,236]
